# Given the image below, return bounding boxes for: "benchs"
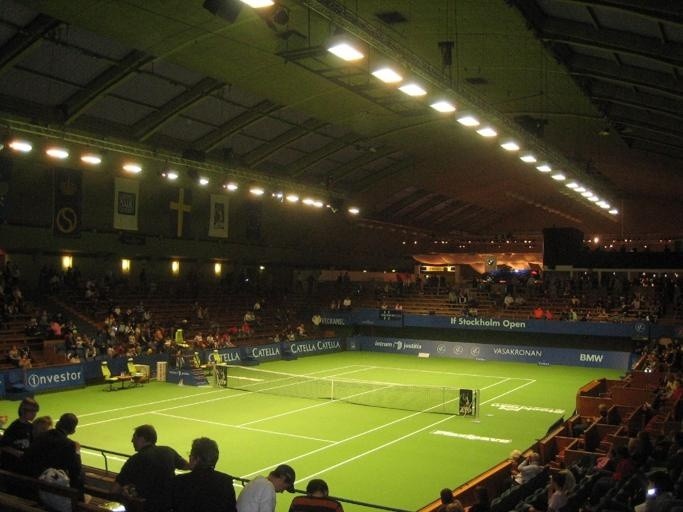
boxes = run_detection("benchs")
[1,428,183,512]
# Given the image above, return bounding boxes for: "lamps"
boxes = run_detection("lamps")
[429,100,456,113]
[456,114,480,127]
[551,174,566,181]
[248,183,361,216]
[519,156,537,163]
[327,42,364,62]
[241,1,275,9]
[398,82,428,97]
[535,165,552,173]
[371,66,404,84]
[475,127,498,137]
[500,142,520,152]
[565,182,619,216]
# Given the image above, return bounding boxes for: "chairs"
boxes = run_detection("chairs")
[272,267,682,348]
[2,269,272,393]
[429,348,682,512]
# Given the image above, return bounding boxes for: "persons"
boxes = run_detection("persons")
[468,486,489,512]
[192,296,265,348]
[287,479,345,511]
[16,414,92,504]
[438,488,465,512]
[2,260,193,371]
[373,267,683,326]
[296,272,318,288]
[0,396,40,472]
[272,305,322,343]
[29,412,53,432]
[507,334,683,511]
[0,415,9,437]
[109,425,190,511]
[337,272,363,297]
[330,295,353,313]
[168,438,238,511]
[235,464,295,511]
